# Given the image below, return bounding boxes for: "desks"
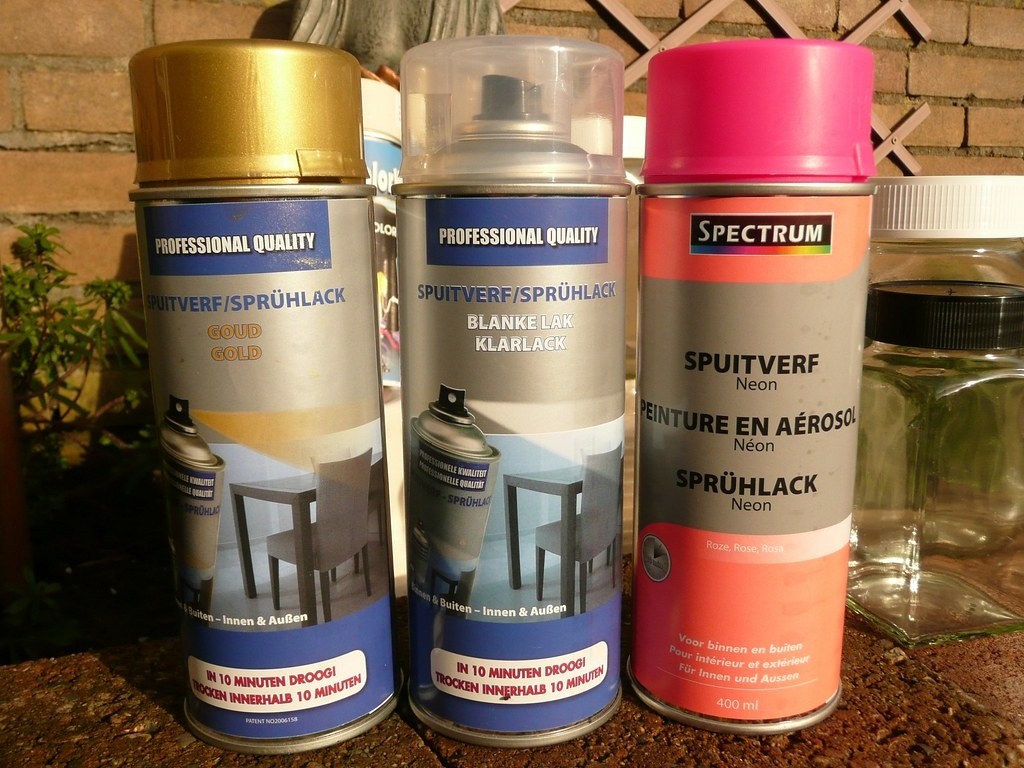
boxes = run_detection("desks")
[503,455,624,619]
[229,451,386,628]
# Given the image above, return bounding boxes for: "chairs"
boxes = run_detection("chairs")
[267,447,373,622]
[535,441,623,614]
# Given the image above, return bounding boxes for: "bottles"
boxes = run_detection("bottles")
[129,39,405,755]
[846,279,1024,650]
[866,175,1024,555]
[636,39,878,736]
[390,36,630,749]
